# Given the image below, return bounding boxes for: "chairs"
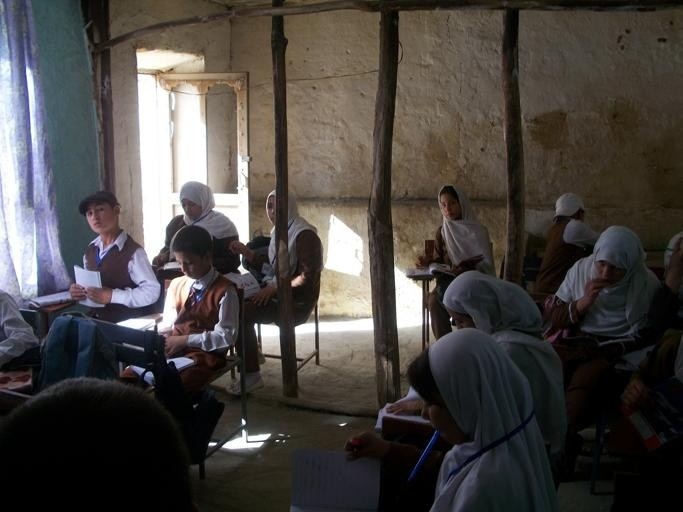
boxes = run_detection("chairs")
[145,277,249,484]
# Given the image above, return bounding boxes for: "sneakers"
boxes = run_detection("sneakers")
[256,347,266,365]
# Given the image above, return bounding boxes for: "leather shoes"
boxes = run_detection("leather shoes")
[225,378,265,396]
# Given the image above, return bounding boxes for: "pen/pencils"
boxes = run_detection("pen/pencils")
[154,324,158,332]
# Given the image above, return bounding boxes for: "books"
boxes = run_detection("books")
[30,290,72,308]
[72,263,107,309]
[406,263,456,278]
[223,272,261,299]
[120,356,195,385]
[290,449,379,511]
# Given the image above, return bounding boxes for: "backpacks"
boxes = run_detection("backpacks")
[32,311,117,392]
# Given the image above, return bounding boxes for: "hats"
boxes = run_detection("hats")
[78,190,119,217]
[550,192,587,223]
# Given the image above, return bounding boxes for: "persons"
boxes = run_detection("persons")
[416,184,496,341]
[541,224,661,417]
[610,232,683,512]
[0,288,40,371]
[152,182,241,281]
[47,191,161,322]
[345,328,561,512]
[226,188,323,395]
[387,270,568,490]
[119,224,241,389]
[528,190,598,304]
